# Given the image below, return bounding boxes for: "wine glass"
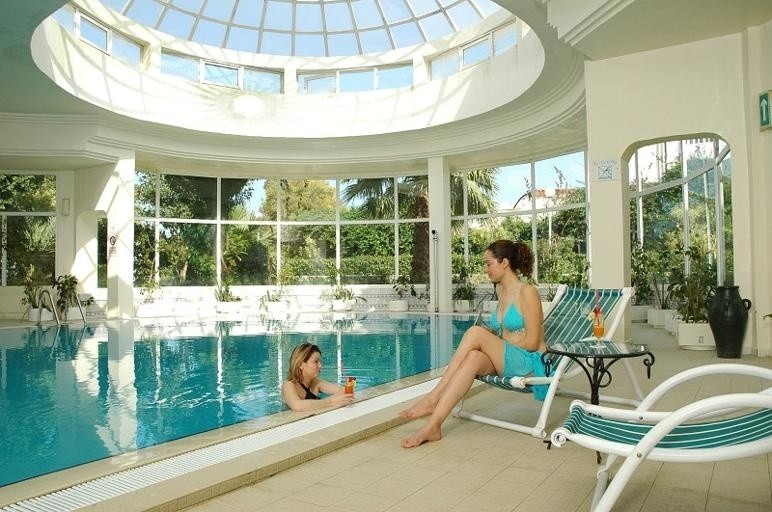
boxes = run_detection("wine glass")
[345,384,354,408]
[589,313,609,349]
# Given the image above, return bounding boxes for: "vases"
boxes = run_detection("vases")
[704,285,752,359]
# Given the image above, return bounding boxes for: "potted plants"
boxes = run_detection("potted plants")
[388,313,493,332]
[20,273,95,322]
[21,324,84,362]
[389,266,507,312]
[320,287,367,311]
[214,279,243,314]
[322,313,367,335]
[213,314,243,339]
[259,289,290,312]
[631,238,725,350]
[261,313,289,333]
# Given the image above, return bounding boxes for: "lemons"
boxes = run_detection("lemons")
[588,313,595,321]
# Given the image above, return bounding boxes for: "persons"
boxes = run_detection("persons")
[397,237,559,450]
[279,342,357,414]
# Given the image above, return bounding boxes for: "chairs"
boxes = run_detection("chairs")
[550,361,772,511]
[451,283,645,439]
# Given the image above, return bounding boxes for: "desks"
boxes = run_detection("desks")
[542,337,655,462]
